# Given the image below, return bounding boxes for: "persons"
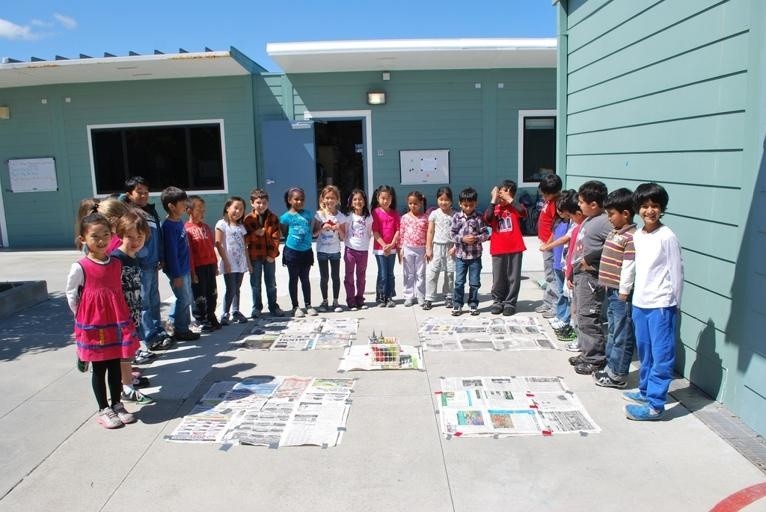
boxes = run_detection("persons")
[533,169,684,422]
[450,186,489,318]
[342,189,372,311]
[213,196,254,325]
[423,186,455,310]
[483,179,528,315]
[313,185,346,312]
[277,186,317,317]
[368,185,399,308]
[64,177,221,430]
[397,191,430,307]
[241,188,284,317]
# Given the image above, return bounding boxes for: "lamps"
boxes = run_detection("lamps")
[0,106,10,120]
[368,93,386,104]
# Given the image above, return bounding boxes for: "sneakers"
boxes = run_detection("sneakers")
[622,392,649,405]
[566,341,627,389]
[318,299,368,312]
[492,306,515,316]
[377,297,432,310]
[95,407,122,429]
[251,305,318,317]
[201,311,247,334]
[623,404,665,421]
[130,330,200,365]
[113,402,136,425]
[120,367,154,404]
[535,303,578,341]
[445,298,480,316]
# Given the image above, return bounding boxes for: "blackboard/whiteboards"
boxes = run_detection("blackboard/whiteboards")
[398,148,451,185]
[7,156,59,194]
